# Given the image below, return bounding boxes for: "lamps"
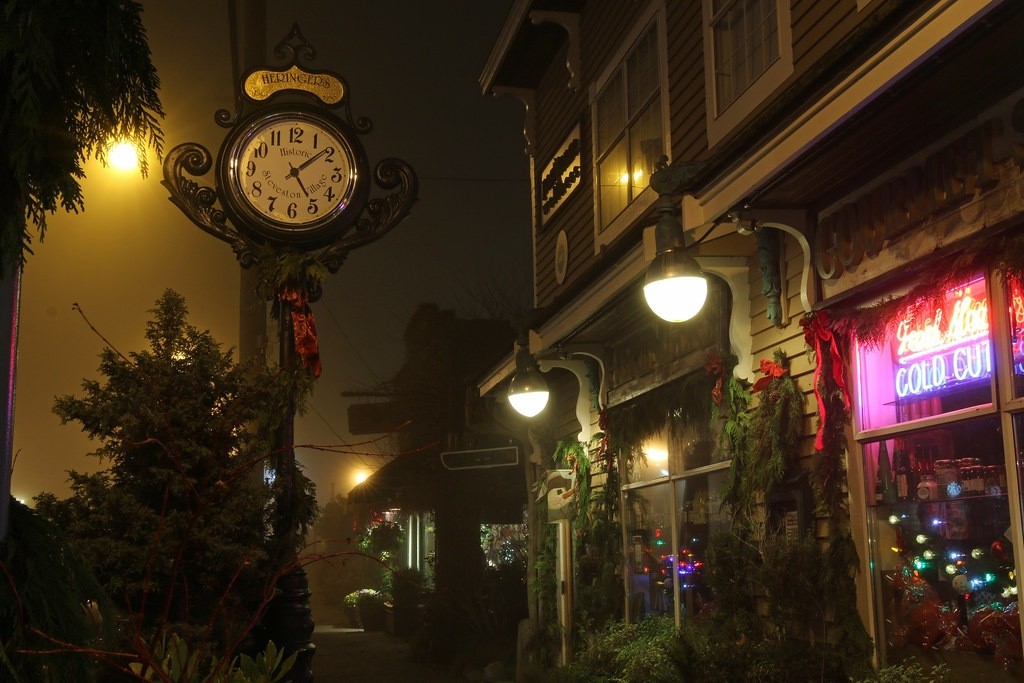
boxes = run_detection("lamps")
[509,306,551,419]
[642,153,711,323]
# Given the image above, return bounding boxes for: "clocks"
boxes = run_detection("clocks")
[215,103,372,253]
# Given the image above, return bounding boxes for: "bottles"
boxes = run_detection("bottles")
[893,450,915,502]
[876,441,896,506]
[916,442,1007,501]
